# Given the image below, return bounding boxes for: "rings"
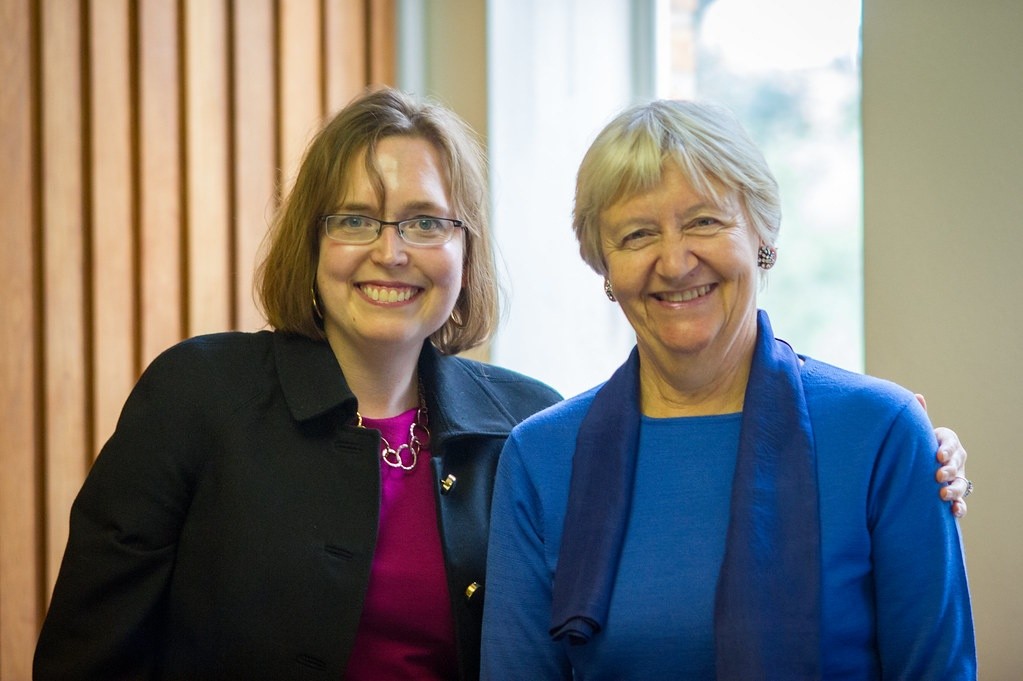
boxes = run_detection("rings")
[954,477,973,499]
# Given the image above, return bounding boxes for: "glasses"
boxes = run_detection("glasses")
[320,214,469,247]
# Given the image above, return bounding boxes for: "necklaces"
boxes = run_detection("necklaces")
[357,368,431,469]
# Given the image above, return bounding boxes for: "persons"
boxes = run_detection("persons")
[33,84,973,681]
[479,100,978,681]
